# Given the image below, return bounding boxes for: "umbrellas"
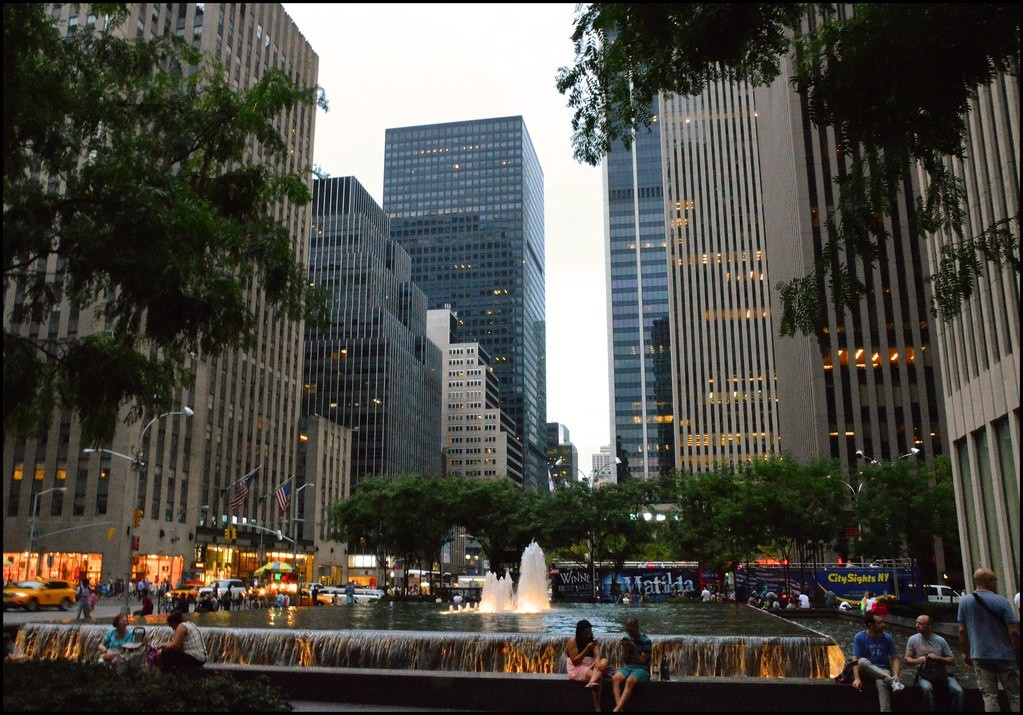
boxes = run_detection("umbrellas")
[253,561,295,576]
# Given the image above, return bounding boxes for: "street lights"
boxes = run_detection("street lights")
[80,407,192,618]
[562,462,621,561]
[25,486,114,586]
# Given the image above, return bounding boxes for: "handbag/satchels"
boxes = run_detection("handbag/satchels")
[920,655,947,685]
[159,649,185,673]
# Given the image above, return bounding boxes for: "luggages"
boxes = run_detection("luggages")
[117,627,147,680]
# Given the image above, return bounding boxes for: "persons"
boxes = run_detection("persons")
[1014,592,1020,617]
[563,585,966,714]
[71,577,482,676]
[957,568,1019,711]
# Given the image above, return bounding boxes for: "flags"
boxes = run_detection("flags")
[229,468,260,510]
[275,479,291,513]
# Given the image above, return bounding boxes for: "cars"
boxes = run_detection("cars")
[164,585,197,602]
[198,578,249,607]
[3,579,77,612]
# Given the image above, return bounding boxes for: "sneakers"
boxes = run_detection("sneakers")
[892,681,905,693]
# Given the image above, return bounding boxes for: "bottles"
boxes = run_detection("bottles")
[653,665,659,680]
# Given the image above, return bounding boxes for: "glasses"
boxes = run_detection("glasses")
[992,578,998,581]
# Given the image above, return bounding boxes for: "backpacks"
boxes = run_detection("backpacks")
[75,585,90,602]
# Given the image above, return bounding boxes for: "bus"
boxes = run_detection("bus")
[425,571,451,589]
